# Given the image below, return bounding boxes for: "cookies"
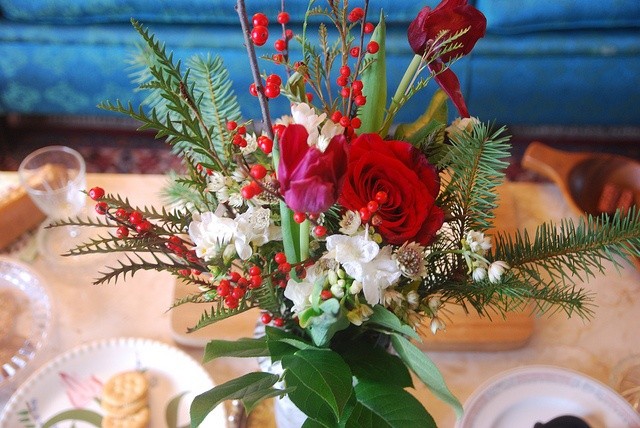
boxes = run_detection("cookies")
[101,371,148,428]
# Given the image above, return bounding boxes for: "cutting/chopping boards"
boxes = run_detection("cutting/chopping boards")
[171,171,537,352]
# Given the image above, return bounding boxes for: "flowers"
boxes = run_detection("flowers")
[43,3,639,428]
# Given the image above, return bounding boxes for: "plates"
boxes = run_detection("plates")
[0,255,53,386]
[453,365,639,428]
[0,338,228,428]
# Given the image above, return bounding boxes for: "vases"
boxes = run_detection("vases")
[268,345,364,427]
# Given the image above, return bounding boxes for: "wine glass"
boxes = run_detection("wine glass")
[18,145,114,270]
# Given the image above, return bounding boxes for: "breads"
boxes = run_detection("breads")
[1,164,68,253]
